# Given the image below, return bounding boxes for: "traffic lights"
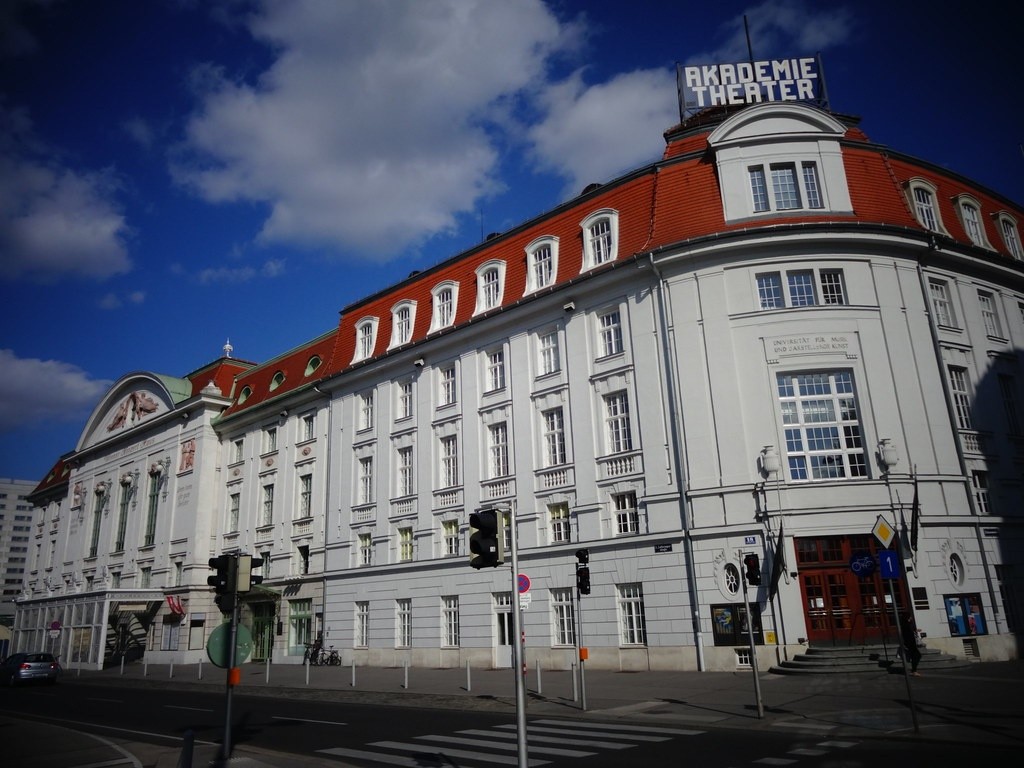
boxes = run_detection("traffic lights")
[576,549,590,595]
[207,554,237,610]
[468,506,503,571]
[745,554,761,585]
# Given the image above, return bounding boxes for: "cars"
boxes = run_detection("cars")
[1,652,57,683]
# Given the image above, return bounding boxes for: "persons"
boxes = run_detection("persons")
[310,630,323,664]
[902,610,924,678]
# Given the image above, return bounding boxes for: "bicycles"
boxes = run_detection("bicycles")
[54,655,63,676]
[301,636,339,667]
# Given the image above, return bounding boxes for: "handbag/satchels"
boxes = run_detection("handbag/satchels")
[913,631,927,647]
[896,644,910,662]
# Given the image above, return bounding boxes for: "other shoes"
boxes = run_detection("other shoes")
[912,674,921,677]
